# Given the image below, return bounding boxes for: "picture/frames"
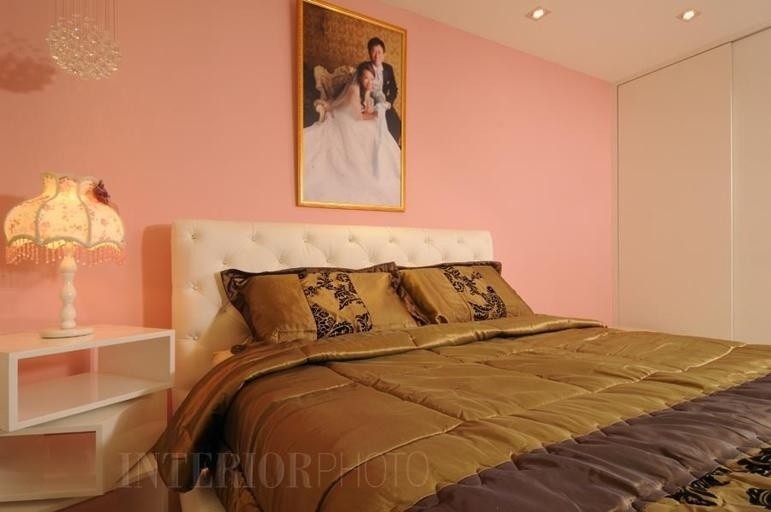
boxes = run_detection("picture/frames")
[296,0,407,212]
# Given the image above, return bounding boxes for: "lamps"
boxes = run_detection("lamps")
[4,172,126,339]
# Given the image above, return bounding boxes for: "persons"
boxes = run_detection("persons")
[321,60,392,204]
[356,37,404,149]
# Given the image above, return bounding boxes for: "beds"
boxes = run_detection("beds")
[172,220,768,512]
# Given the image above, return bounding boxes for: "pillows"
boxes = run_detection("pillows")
[221,262,430,344]
[398,261,537,324]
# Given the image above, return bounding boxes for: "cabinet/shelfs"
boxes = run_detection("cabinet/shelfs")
[0,322,176,511]
